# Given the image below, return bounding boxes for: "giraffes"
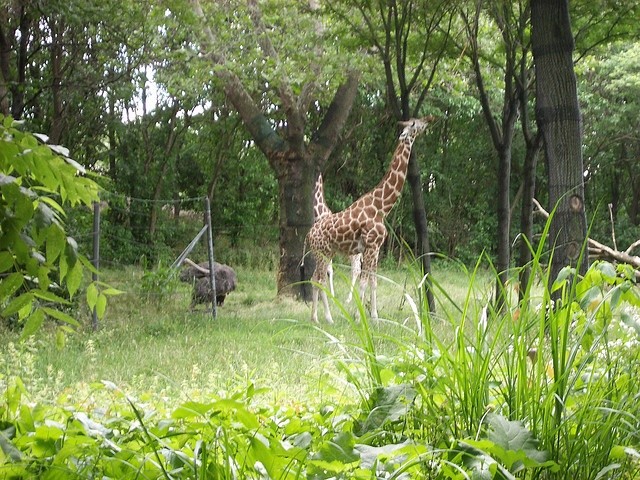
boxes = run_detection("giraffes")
[314,171,365,305]
[302,113,435,324]
